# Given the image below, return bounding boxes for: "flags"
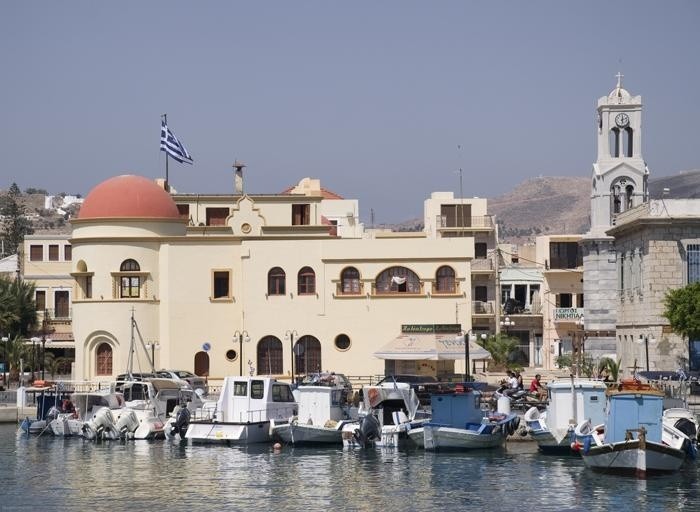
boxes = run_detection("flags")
[160,120,193,164]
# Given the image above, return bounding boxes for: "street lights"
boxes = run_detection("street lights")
[2,337,9,385]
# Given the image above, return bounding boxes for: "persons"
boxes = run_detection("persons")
[530,375,548,401]
[502,367,523,401]
[63,395,75,413]
